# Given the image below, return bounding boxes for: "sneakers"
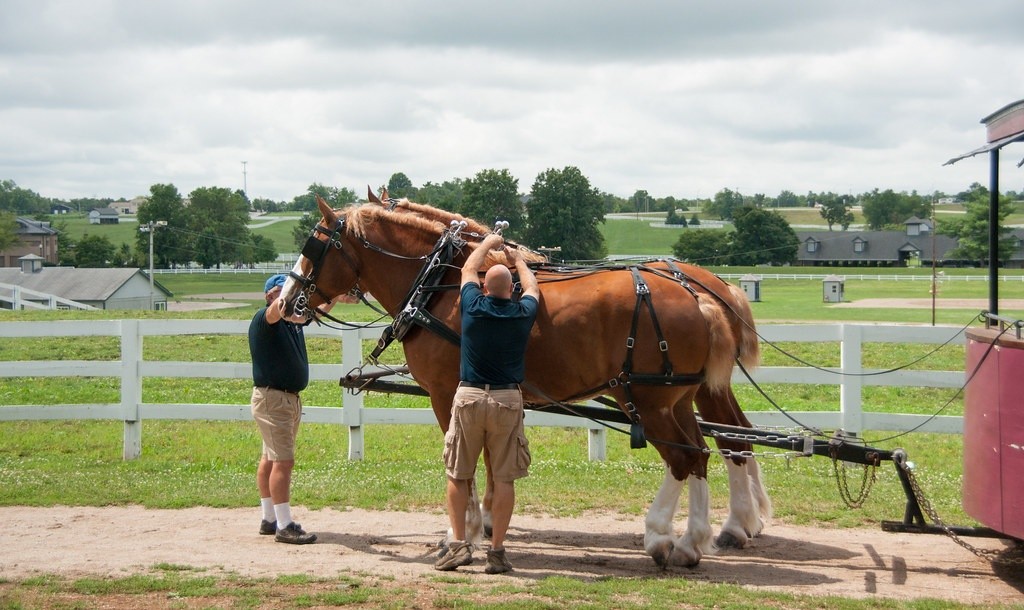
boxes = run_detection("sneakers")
[486,547,513,574]
[435,542,473,570]
[259,520,318,545]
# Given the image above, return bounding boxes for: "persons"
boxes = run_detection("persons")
[248,274,362,545]
[435,234,540,573]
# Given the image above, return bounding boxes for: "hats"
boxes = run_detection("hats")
[264,274,289,293]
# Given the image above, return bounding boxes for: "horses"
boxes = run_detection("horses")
[278,184,774,572]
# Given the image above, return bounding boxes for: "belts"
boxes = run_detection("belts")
[460,381,520,390]
[255,385,296,394]
